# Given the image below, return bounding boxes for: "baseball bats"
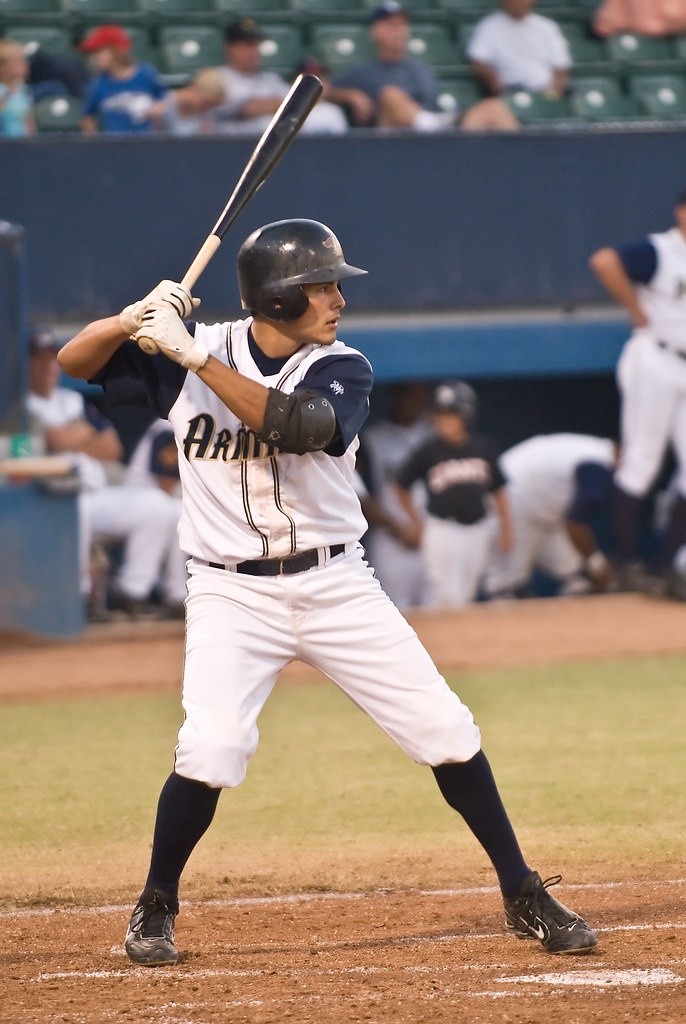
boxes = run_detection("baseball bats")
[138,72,325,354]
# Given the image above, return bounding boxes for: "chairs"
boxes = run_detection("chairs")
[0,0,686,136]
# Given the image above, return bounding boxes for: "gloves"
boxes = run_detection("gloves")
[130,301,210,374]
[120,280,201,335]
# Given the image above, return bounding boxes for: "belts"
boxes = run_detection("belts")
[657,338,686,360]
[209,540,344,576]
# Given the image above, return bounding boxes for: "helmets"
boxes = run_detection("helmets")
[432,380,475,416]
[237,218,367,321]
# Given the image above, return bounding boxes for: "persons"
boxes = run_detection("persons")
[596,0,686,37]
[468,0,573,99]
[81,27,167,135]
[295,58,347,129]
[56,218,596,965]
[208,18,289,130]
[1,41,37,137]
[328,0,517,130]
[590,190,686,598]
[32,325,189,619]
[152,70,225,133]
[354,378,622,607]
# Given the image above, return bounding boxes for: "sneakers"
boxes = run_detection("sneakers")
[124,890,179,963]
[505,870,599,955]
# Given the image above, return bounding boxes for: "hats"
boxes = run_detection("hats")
[222,17,266,42]
[368,0,405,25]
[28,321,58,355]
[77,23,130,54]
